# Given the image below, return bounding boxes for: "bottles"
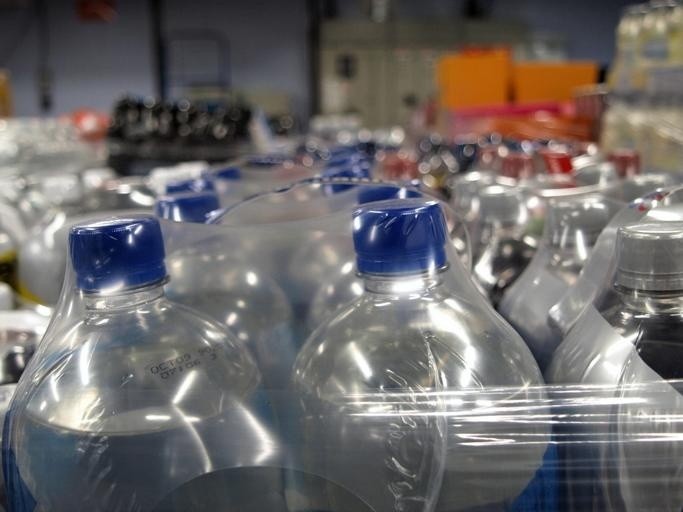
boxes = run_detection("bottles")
[595,0,683,177]
[1,90,683,512]
